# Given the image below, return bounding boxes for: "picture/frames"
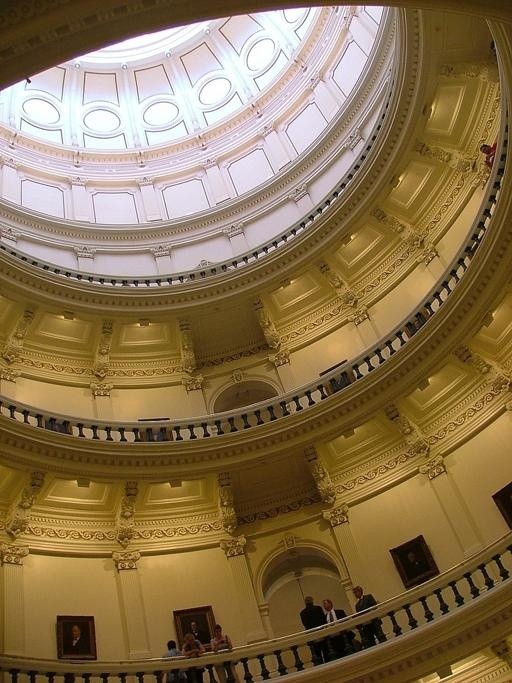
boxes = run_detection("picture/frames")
[56,612,98,665]
[171,604,221,653]
[491,479,511,532]
[388,533,441,590]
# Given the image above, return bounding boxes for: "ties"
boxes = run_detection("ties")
[329,611,334,622]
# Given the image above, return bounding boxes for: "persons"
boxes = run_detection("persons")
[64,622,91,654]
[210,622,238,682]
[182,633,207,682]
[352,584,383,645]
[299,595,330,665]
[162,639,189,682]
[322,598,355,658]
[405,550,427,581]
[479,141,497,169]
[188,618,211,644]
